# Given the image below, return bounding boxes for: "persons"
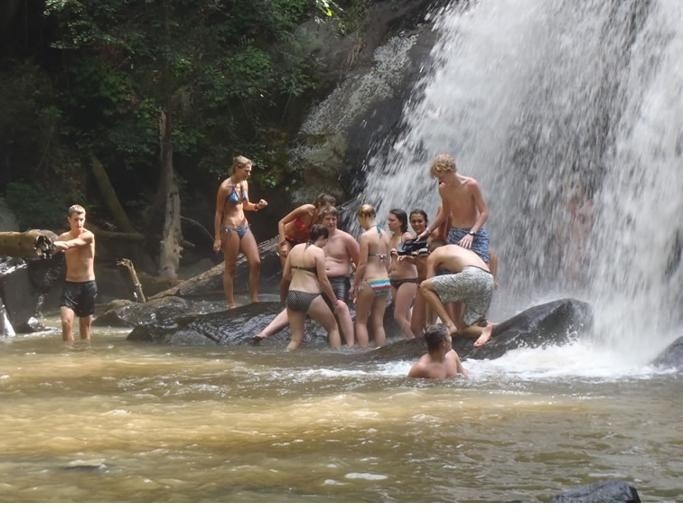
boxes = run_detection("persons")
[252,192,497,353]
[49,204,98,342]
[403,323,473,382]
[214,154,268,309]
[416,154,491,266]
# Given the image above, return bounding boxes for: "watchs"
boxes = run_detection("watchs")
[468,230,474,235]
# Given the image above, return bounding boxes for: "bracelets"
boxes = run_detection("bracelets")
[426,228,431,234]
[254,203,258,211]
